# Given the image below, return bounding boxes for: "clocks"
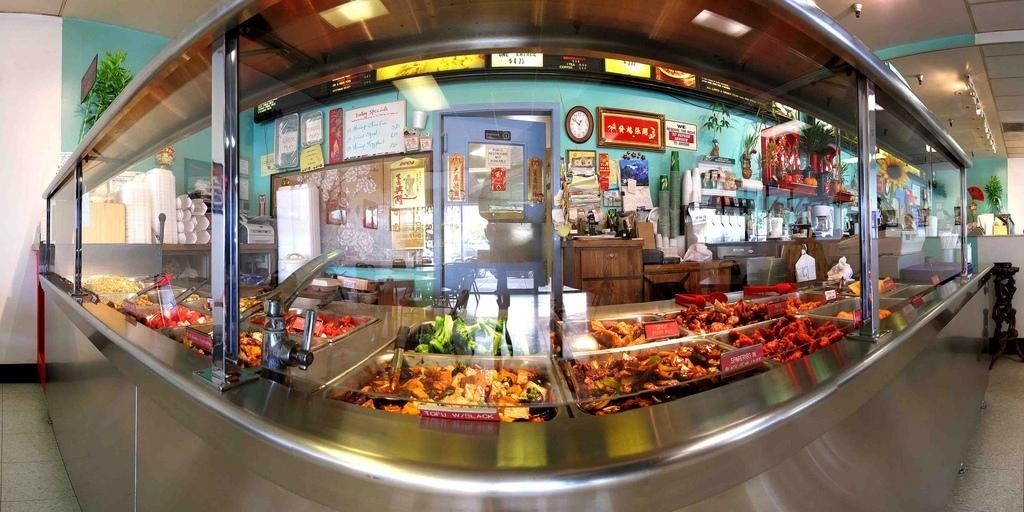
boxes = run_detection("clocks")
[565,105,594,144]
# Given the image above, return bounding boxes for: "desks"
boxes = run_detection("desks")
[644,260,734,301]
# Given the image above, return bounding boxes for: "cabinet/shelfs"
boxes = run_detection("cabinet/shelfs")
[26,263,996,511]
[562,240,643,307]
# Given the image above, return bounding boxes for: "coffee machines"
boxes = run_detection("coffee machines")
[812,206,834,238]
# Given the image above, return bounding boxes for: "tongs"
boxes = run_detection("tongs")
[674,292,728,307]
[744,283,798,295]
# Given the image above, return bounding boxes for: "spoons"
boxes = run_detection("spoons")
[383,327,410,399]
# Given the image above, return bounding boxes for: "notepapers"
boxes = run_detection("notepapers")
[558,224,570,242]
[628,178,637,195]
[552,208,564,231]
[554,190,563,206]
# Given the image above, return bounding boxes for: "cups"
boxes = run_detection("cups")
[669,150,680,237]
[659,174,669,237]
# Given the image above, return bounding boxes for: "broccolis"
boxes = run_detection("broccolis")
[418,315,528,358]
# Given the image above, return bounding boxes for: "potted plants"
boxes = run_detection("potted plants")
[984,176,1003,214]
[740,136,757,179]
[832,162,848,193]
[703,102,731,157]
[802,122,837,174]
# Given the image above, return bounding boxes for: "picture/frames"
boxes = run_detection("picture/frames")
[566,150,596,174]
[596,106,667,154]
